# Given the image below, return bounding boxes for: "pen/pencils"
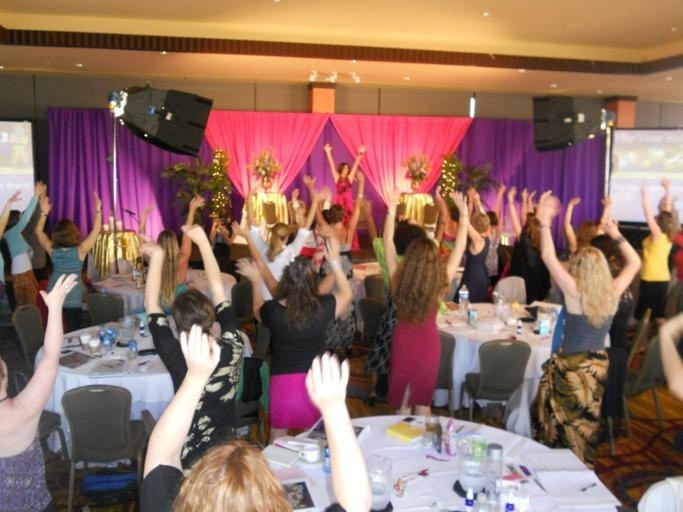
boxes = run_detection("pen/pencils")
[435,423,442,453]
[456,426,464,434]
[582,483,596,491]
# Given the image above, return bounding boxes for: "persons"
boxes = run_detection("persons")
[1,272,79,511]
[1,183,247,468]
[228,173,470,440]
[140,319,372,511]
[324,144,366,252]
[451,177,683,471]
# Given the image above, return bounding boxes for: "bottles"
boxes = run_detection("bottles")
[465,443,517,512]
[516,320,523,334]
[458,284,470,316]
[131,256,148,289]
[98,321,145,353]
[323,443,331,474]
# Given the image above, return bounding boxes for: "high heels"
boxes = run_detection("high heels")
[363,393,376,408]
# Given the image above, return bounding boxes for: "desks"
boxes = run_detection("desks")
[243,190,290,234]
[395,192,434,238]
[88,228,145,278]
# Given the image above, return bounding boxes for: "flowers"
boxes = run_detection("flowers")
[401,154,431,180]
[245,149,283,179]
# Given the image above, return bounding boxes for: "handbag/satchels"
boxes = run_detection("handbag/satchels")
[83,468,138,506]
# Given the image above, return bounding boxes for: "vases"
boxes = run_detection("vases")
[260,179,271,194]
[411,179,422,194]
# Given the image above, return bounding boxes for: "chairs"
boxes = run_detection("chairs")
[11,293,175,512]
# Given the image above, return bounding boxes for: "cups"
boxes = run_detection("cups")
[80,332,92,350]
[88,337,101,353]
[457,453,486,492]
[365,454,394,512]
[299,444,320,462]
